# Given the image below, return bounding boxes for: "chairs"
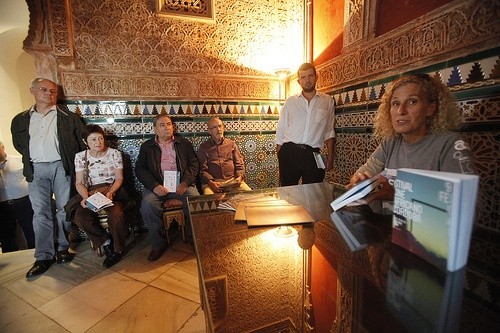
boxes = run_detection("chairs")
[162,198,186,249]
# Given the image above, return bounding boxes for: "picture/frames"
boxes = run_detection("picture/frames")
[156,0,215,22]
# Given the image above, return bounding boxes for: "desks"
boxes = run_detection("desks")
[185,182,500,333]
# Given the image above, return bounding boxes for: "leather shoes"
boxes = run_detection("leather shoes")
[56,250,73,264]
[26,259,56,279]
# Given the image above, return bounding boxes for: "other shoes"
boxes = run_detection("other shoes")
[103,252,126,268]
[148,245,168,261]
[103,238,114,257]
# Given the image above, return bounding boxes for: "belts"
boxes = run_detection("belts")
[288,142,318,152]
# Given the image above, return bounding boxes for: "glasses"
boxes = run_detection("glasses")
[34,87,58,94]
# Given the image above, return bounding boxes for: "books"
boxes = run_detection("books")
[86,192,114,212]
[220,182,239,188]
[164,170,180,193]
[218,194,315,226]
[391,168,479,272]
[331,211,379,252]
[330,169,388,211]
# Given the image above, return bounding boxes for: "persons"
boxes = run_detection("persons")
[274,63,334,187]
[345,74,476,206]
[198,117,252,195]
[11,77,82,279]
[75,124,129,267]
[135,114,199,260]
